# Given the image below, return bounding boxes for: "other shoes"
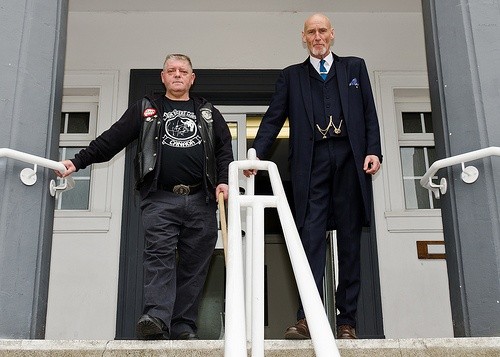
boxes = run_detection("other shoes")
[136,313,170,340]
[163,322,198,339]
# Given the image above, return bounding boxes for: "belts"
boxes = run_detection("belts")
[157,182,203,196]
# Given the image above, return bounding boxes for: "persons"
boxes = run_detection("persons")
[55,54,235,340]
[243,14,383,339]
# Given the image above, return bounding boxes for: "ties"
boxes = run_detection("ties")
[319,59,328,80]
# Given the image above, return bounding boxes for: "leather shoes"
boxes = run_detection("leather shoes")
[284,319,311,339]
[337,324,359,339]
